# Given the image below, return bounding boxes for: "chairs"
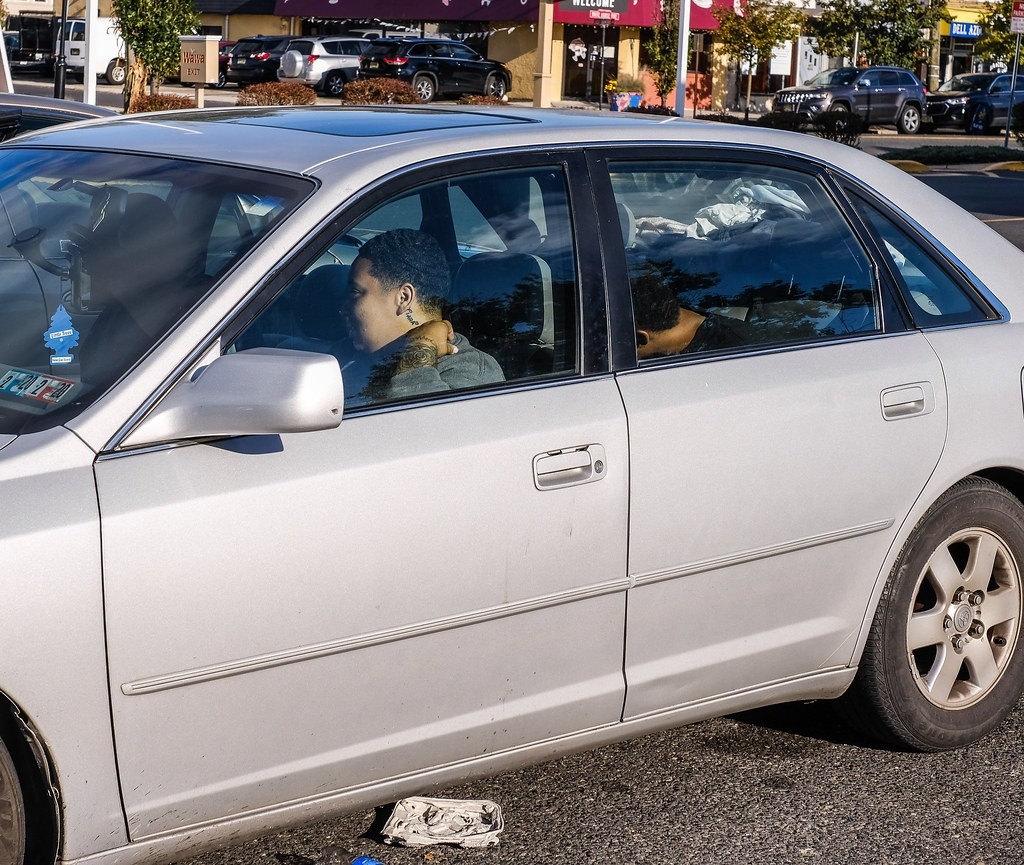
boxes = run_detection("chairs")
[445,252,555,377]
[273,261,353,364]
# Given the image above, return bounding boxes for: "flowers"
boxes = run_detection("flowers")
[603,74,645,96]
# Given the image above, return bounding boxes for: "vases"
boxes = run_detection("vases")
[606,92,642,112]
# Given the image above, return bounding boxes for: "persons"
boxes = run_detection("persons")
[631,262,805,358]
[856,56,868,67]
[319,229,506,407]
[60,194,266,389]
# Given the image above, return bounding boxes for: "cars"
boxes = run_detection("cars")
[929,72,1024,135]
[359,36,515,105]
[55,15,141,83]
[224,34,293,89]
[1,15,59,76]
[772,65,930,136]
[0,108,1023,864]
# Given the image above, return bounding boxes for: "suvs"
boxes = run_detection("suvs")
[275,34,366,97]
